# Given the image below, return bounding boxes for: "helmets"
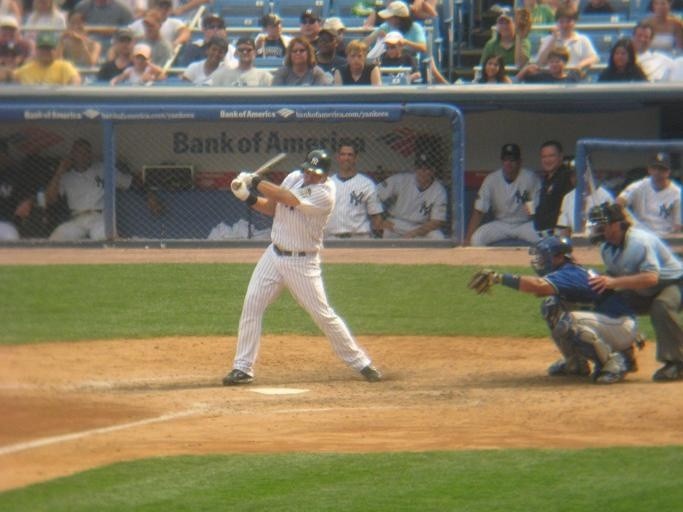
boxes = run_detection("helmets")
[529,236,576,276]
[588,202,633,245]
[302,150,332,175]
[501,143,520,160]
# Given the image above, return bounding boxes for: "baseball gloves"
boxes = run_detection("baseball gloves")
[468,268,500,294]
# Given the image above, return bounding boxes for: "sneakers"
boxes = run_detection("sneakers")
[549,362,589,377]
[653,364,683,383]
[596,371,623,384]
[360,365,381,383]
[222,369,255,385]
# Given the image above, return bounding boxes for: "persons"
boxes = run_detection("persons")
[0,135,170,241]
[584,199,683,383]
[1,1,683,84]
[324,141,683,249]
[218,147,384,389]
[465,233,638,384]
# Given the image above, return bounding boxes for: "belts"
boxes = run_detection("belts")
[273,244,306,257]
[70,208,103,217]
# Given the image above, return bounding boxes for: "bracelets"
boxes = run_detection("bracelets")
[495,271,521,291]
[244,192,258,207]
[252,176,262,190]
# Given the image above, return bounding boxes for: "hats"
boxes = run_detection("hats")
[648,152,673,171]
[415,150,434,168]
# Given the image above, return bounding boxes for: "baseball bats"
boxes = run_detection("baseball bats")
[233,153,287,189]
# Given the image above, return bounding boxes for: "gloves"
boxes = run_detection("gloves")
[230,178,257,206]
[238,172,264,194]
